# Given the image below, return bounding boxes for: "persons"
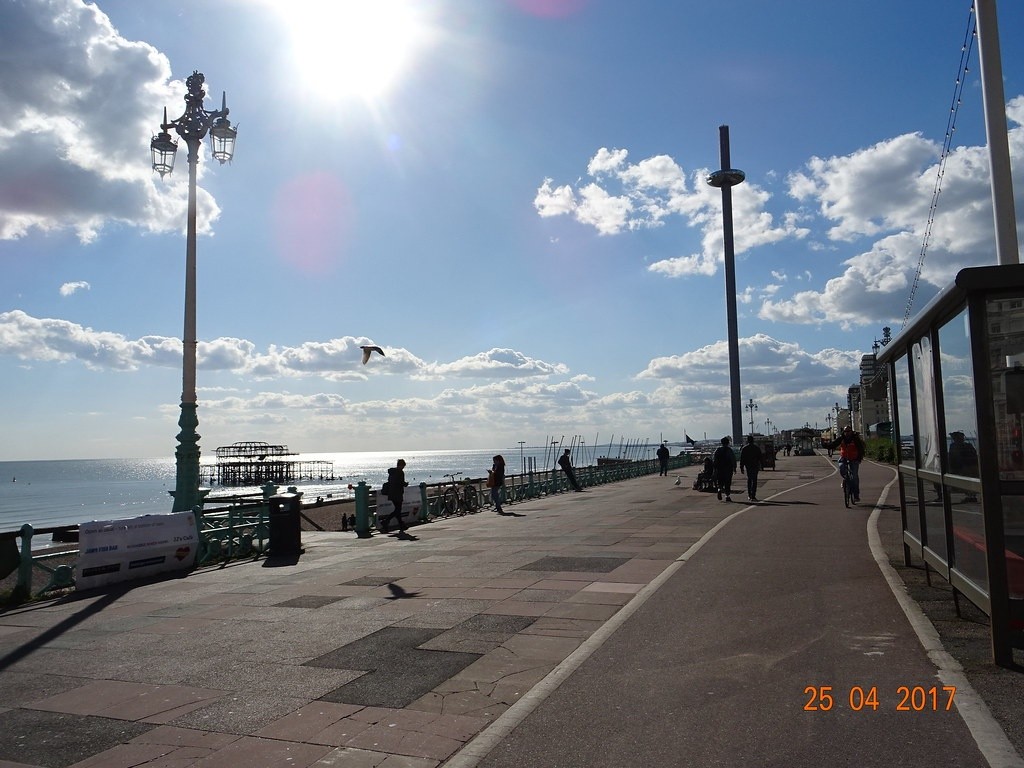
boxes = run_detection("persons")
[342,513,357,530]
[784,442,792,456]
[821,425,865,502]
[657,444,669,476]
[558,449,583,492]
[380,459,409,532]
[487,455,505,515]
[691,435,762,502]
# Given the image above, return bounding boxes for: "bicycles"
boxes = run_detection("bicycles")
[443,471,478,514]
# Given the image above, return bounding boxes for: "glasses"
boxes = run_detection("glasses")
[844,430,850,432]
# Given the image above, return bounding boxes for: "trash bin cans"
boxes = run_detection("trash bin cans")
[268,492,301,558]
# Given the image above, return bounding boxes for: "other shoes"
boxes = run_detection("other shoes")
[725,497,731,501]
[400,525,409,532]
[854,497,859,502]
[748,496,756,500]
[717,490,723,501]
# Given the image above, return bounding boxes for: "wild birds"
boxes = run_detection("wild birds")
[674,476,681,486]
[359,345,386,365]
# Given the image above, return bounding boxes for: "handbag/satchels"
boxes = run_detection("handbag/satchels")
[486,473,494,488]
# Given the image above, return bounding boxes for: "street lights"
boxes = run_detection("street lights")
[151,70,238,562]
[825,402,842,444]
[764,418,777,440]
[745,397,759,440]
